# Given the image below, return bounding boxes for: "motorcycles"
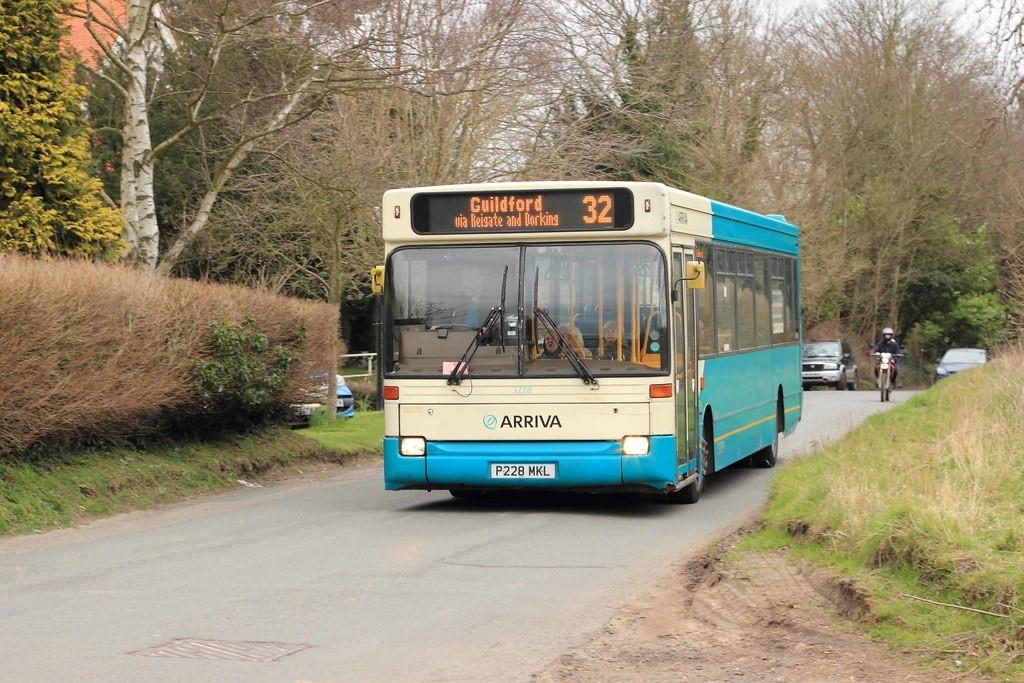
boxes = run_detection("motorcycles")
[868,351,904,404]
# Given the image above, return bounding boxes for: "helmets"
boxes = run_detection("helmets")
[882,327,894,335]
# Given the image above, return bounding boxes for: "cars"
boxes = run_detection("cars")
[286,369,356,423]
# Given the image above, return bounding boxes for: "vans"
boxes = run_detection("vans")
[935,348,989,385]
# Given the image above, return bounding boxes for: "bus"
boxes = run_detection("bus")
[369,182,805,503]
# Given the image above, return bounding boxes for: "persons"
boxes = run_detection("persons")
[536,273,799,362]
[870,327,902,389]
[428,261,500,347]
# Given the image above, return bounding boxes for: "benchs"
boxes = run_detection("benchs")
[573,304,661,361]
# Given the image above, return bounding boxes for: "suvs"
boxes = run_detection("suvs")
[802,337,860,392]
